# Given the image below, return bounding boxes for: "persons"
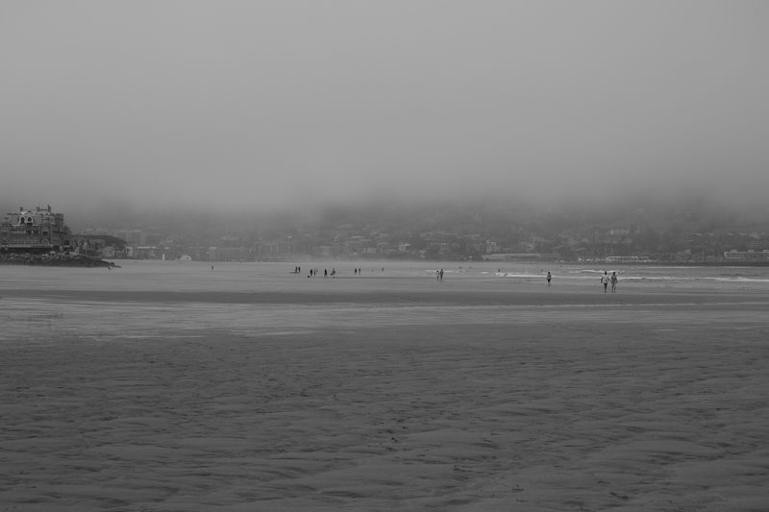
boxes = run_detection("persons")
[436,268,443,280]
[601,271,618,293]
[546,272,551,285]
[354,268,361,275]
[295,266,336,279]
